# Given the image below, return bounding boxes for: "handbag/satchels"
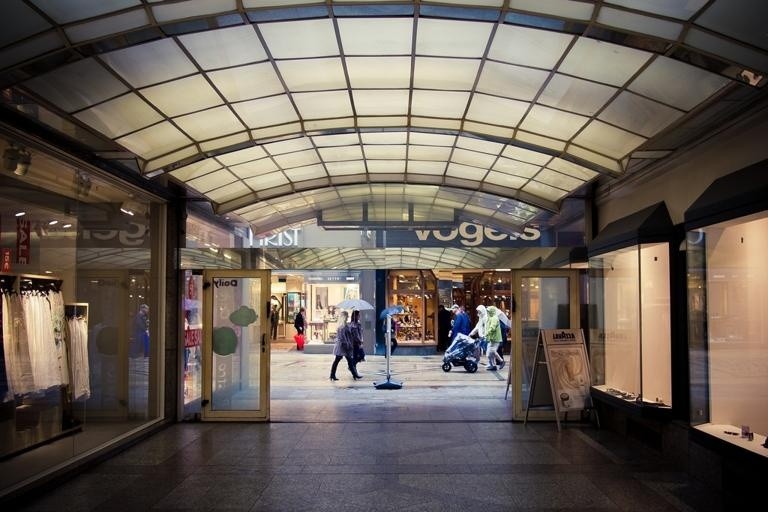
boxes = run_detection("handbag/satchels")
[353,348,365,365]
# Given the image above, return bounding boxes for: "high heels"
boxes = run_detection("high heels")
[330,376,338,381]
[353,375,362,380]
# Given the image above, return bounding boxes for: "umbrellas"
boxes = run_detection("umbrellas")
[495,309,511,329]
[380,305,406,319]
[334,299,375,311]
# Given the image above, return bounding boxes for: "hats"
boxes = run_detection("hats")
[450,304,459,310]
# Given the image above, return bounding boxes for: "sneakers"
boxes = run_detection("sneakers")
[486,358,506,371]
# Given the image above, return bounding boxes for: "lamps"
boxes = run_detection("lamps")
[2,140,31,179]
[120,200,146,219]
[72,169,93,199]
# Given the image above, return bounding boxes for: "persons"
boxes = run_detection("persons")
[269,305,279,340]
[348,310,365,371]
[436,304,508,371]
[130,304,148,358]
[184,309,192,378]
[294,308,305,350]
[383,317,398,359]
[329,308,363,381]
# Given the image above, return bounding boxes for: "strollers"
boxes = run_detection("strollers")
[442,333,486,373]
